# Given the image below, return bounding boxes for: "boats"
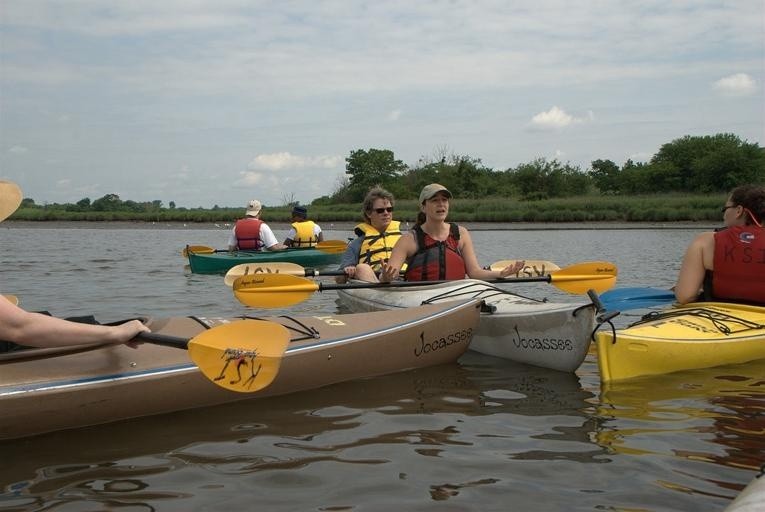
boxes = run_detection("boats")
[0,293,487,447]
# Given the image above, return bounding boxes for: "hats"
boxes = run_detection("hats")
[245,199,262,217]
[419,182,453,204]
[292,206,306,217]
[0,181,24,223]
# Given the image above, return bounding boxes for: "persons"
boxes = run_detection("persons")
[378,183,525,283]
[282,206,323,248]
[227,199,288,253]
[674,183,765,308]
[0,286,151,353]
[334,188,409,284]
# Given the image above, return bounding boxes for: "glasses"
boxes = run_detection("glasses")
[373,206,393,213]
[720,206,735,212]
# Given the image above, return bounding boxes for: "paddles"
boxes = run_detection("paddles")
[182,240,349,261]
[3,295,290,392]
[233,262,618,307]
[597,288,678,311]
